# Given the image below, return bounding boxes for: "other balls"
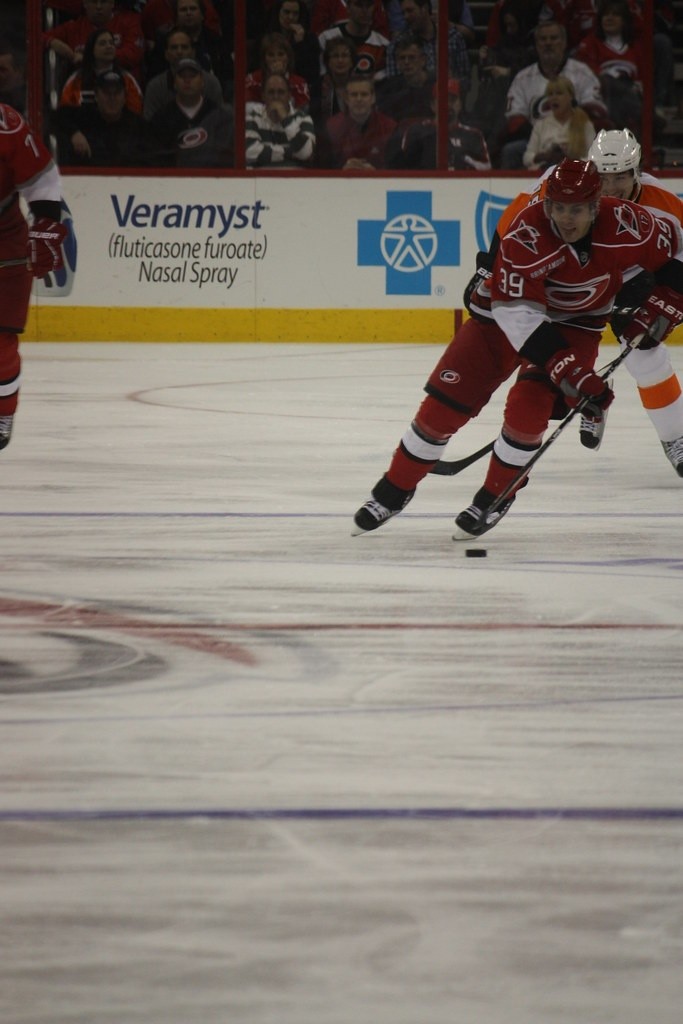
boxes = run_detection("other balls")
[465,549,487,557]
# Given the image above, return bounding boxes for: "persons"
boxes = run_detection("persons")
[464,128,682,480]
[0,0,683,169]
[0,100,64,450]
[349,158,683,543]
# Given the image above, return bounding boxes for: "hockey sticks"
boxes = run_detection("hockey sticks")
[429,359,614,476]
[451,313,651,540]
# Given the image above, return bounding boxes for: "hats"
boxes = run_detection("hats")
[95,71,125,91]
[432,79,459,98]
[175,58,203,77]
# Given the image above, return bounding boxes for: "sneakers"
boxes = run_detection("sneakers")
[451,476,529,541]
[579,373,614,451]
[660,436,683,478]
[0,413,14,451]
[351,472,416,536]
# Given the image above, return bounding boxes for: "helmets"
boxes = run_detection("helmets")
[588,127,641,178]
[543,157,602,207]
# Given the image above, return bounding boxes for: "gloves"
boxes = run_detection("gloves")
[623,283,683,350]
[463,251,495,318]
[545,345,615,418]
[24,218,67,278]
